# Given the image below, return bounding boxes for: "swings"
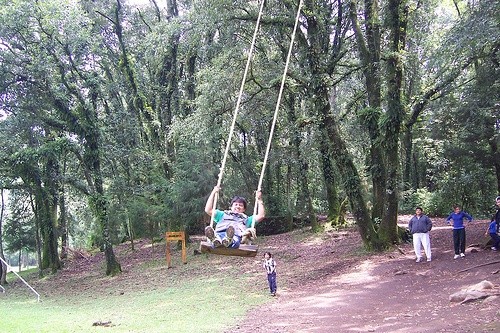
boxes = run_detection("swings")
[199,0,304,257]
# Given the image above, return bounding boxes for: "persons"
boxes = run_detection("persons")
[484,196,500,251]
[263,252,278,296]
[408,207,432,263]
[446,205,472,259]
[205,186,266,249]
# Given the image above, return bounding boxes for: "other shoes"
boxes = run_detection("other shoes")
[205,226,221,248]
[222,226,235,247]
[454,254,459,259]
[492,247,497,251]
[416,259,420,262]
[272,292,276,297]
[427,258,431,262]
[460,253,465,257]
